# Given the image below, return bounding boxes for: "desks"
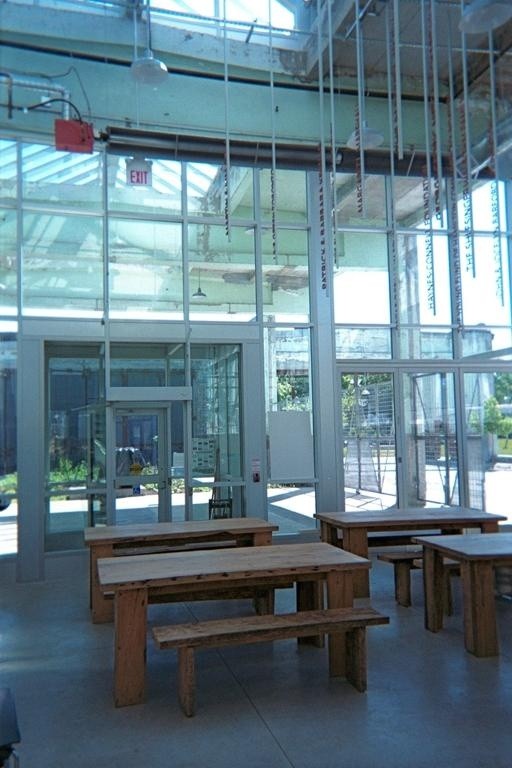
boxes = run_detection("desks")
[313,506,510,607]
[91,541,377,706]
[411,529,512,659]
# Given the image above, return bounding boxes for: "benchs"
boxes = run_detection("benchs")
[378,548,431,608]
[319,530,458,553]
[152,605,390,717]
[83,514,281,625]
[413,556,462,619]
[102,579,298,616]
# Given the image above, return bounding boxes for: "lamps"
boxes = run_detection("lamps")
[191,267,206,302]
[458,0,512,36]
[346,24,385,150]
[127,0,168,88]
